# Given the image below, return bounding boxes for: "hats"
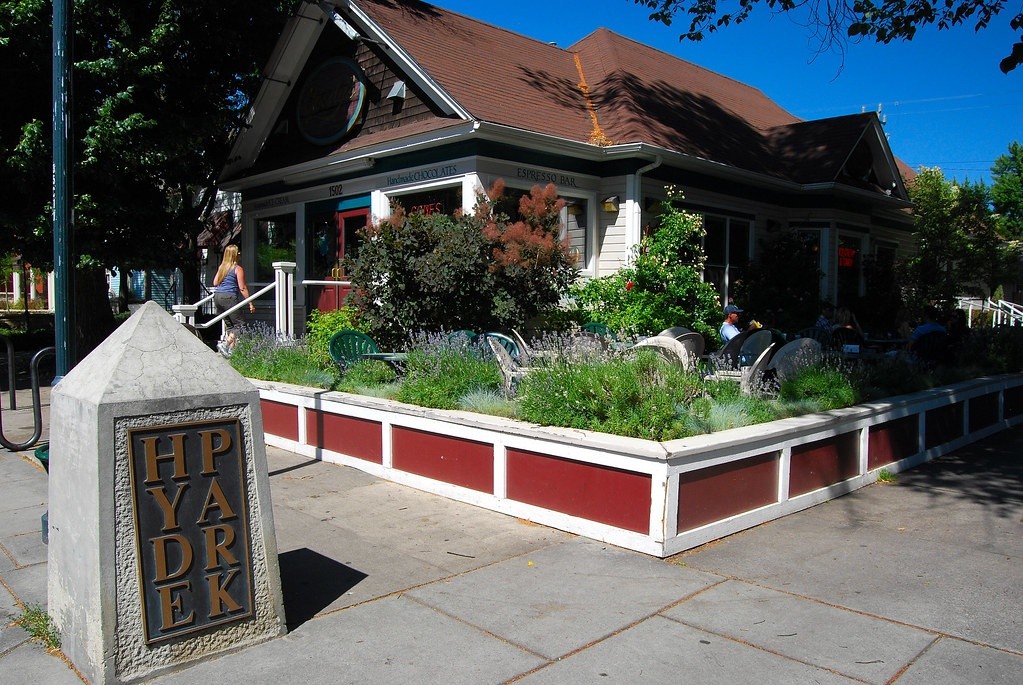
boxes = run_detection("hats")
[724,304,740,314]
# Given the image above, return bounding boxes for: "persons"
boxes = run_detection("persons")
[815,301,833,335]
[833,307,864,336]
[909,306,947,353]
[719,304,754,349]
[213,244,255,357]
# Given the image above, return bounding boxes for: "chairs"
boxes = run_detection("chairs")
[327,322,971,407]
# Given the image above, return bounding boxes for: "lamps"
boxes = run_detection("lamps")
[600,195,619,212]
[567,204,585,215]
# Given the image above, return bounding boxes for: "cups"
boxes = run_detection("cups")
[795,334,801,339]
[864,332,868,340]
